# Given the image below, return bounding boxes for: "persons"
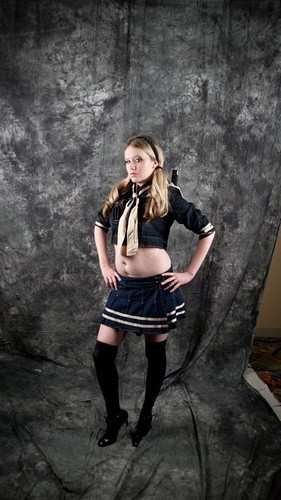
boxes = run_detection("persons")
[92,135,216,448]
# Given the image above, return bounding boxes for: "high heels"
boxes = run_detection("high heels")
[97,410,129,445]
[132,413,153,446]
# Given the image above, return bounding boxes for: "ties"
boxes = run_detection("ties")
[115,181,152,256]
